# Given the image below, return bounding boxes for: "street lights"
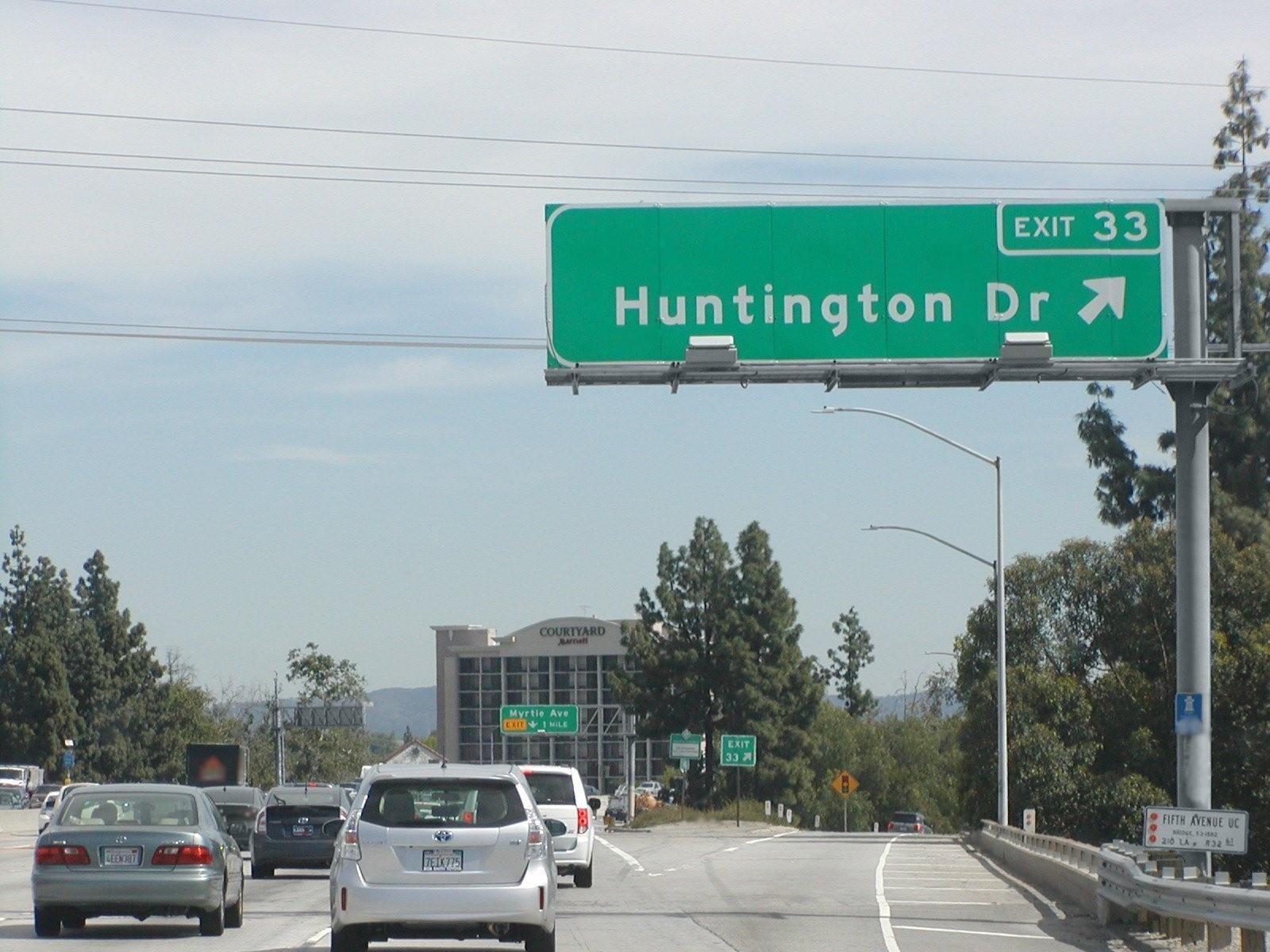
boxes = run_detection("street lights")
[821,404,1008,827]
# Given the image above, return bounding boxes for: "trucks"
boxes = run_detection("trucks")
[0,764,44,792]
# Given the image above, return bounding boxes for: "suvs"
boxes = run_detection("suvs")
[887,811,933,833]
[454,763,604,891]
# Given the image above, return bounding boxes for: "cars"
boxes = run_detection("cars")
[32,745,558,951]
[615,781,662,802]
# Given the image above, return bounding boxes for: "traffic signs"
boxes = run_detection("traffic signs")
[542,200,1172,388]
[721,735,757,767]
[501,706,579,738]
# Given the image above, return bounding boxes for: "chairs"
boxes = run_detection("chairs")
[92,807,119,826]
[476,792,508,825]
[384,791,415,824]
